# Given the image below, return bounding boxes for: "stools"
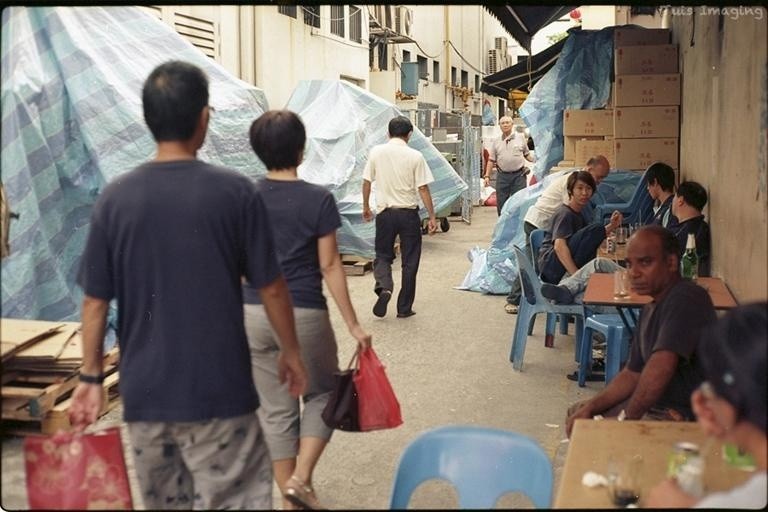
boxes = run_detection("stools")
[579,310,642,384]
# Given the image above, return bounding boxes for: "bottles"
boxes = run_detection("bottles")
[681,232,699,283]
[666,441,706,501]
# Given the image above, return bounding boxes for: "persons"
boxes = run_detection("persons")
[239,106,373,512]
[566,223,719,439]
[479,115,536,217]
[63,55,309,511]
[504,155,711,381]
[360,115,437,319]
[692,300,767,509]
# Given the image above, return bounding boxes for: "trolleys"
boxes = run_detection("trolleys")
[419,152,458,234]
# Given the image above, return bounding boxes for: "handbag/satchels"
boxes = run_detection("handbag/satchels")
[320,350,362,433]
[24,426,132,510]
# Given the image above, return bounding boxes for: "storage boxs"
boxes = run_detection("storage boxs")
[550,28,682,181]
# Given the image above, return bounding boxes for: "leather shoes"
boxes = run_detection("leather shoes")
[541,284,573,304]
[567,371,591,382]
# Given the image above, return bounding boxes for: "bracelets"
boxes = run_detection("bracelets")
[76,373,106,385]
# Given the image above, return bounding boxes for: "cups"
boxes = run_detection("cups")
[605,447,643,505]
[613,271,631,298]
[629,222,646,236]
[616,227,627,245]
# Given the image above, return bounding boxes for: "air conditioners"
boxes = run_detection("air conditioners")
[484,36,513,77]
[371,5,413,37]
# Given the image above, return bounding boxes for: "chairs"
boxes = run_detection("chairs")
[596,160,665,236]
[509,245,588,373]
[387,424,556,512]
[522,227,571,336]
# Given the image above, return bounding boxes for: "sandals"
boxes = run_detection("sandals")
[505,304,518,313]
[283,475,328,511]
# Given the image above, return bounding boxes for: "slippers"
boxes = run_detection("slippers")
[373,291,391,317]
[397,311,416,317]
